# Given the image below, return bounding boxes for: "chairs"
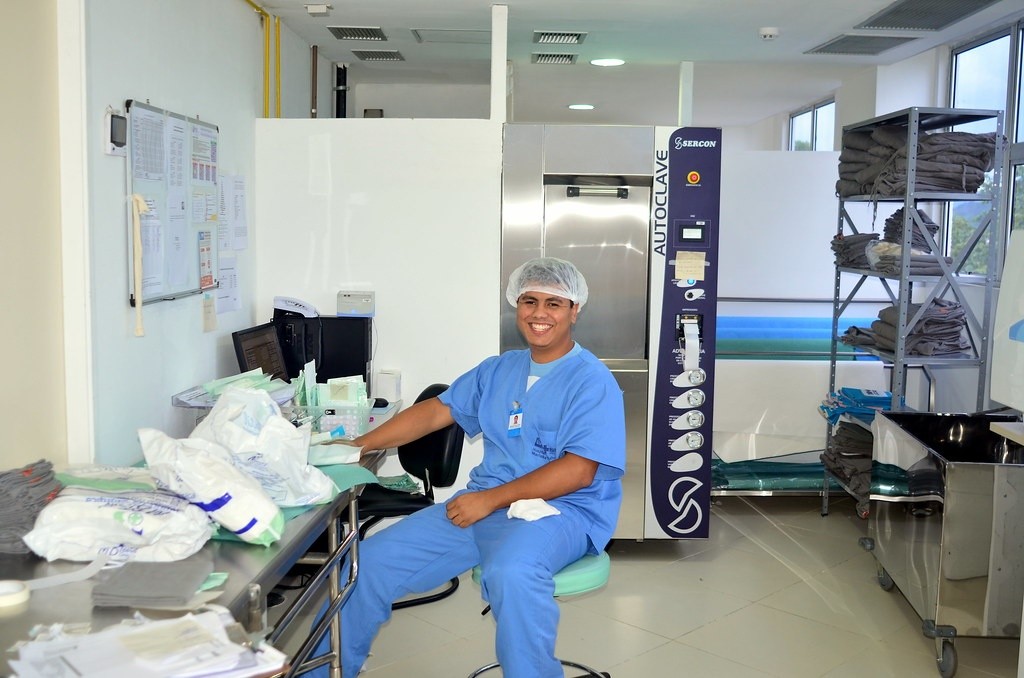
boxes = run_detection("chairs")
[324,377,471,607]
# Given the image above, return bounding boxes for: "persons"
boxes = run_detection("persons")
[290,256,626,678]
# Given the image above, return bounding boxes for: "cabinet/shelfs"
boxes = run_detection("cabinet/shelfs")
[821,105,1005,550]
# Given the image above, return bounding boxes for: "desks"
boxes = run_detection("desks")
[354,401,405,451]
[1,448,388,676]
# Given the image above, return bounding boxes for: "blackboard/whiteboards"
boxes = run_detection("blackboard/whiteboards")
[126,99,220,307]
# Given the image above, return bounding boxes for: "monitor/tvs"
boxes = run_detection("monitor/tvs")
[232,323,292,393]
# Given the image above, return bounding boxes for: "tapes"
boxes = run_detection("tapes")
[0,554,109,606]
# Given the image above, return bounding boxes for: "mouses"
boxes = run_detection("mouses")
[372,398,389,408]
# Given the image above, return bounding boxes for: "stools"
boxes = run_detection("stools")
[466,541,615,678]
